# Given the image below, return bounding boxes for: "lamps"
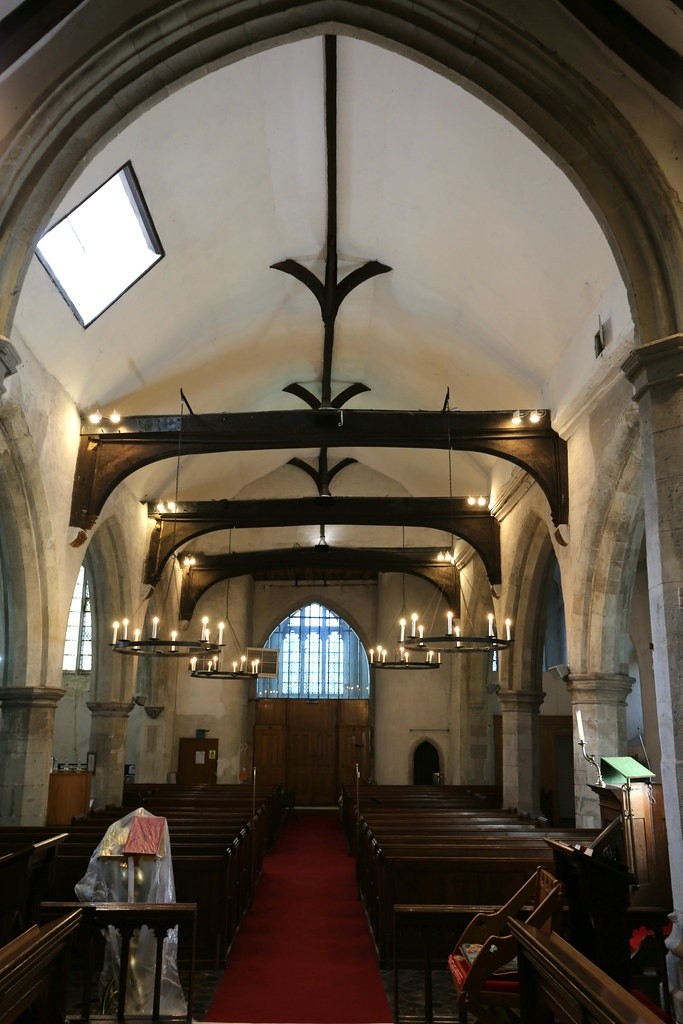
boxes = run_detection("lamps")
[400,393,516,651]
[511,408,541,423]
[369,527,442,669]
[437,550,451,560]
[184,554,196,566]
[467,494,486,507]
[156,497,175,511]
[90,407,120,423]
[112,389,221,660]
[190,529,259,682]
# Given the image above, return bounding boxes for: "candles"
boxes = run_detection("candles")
[576,709,585,740]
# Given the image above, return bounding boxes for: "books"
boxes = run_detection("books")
[123,816,166,857]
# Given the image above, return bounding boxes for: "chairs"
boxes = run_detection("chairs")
[449,866,564,1024]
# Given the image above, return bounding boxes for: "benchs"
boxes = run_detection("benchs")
[46,782,283,957]
[342,779,609,965]
